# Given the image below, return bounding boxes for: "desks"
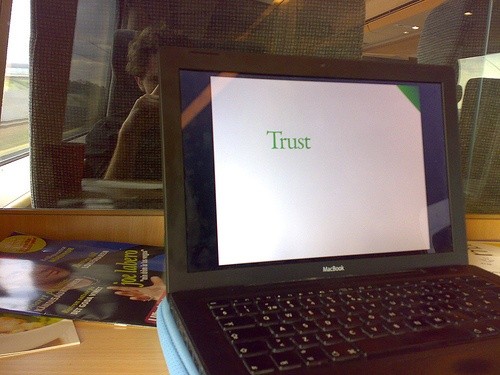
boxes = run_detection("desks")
[0,209,498,375]
[81,176,163,201]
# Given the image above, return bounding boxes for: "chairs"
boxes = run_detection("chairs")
[106,30,267,140]
[455,77,499,214]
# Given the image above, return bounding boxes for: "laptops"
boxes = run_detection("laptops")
[158,44,500,375]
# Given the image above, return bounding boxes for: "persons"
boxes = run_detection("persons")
[0,230,164,327]
[2,258,166,316]
[85,24,187,180]
